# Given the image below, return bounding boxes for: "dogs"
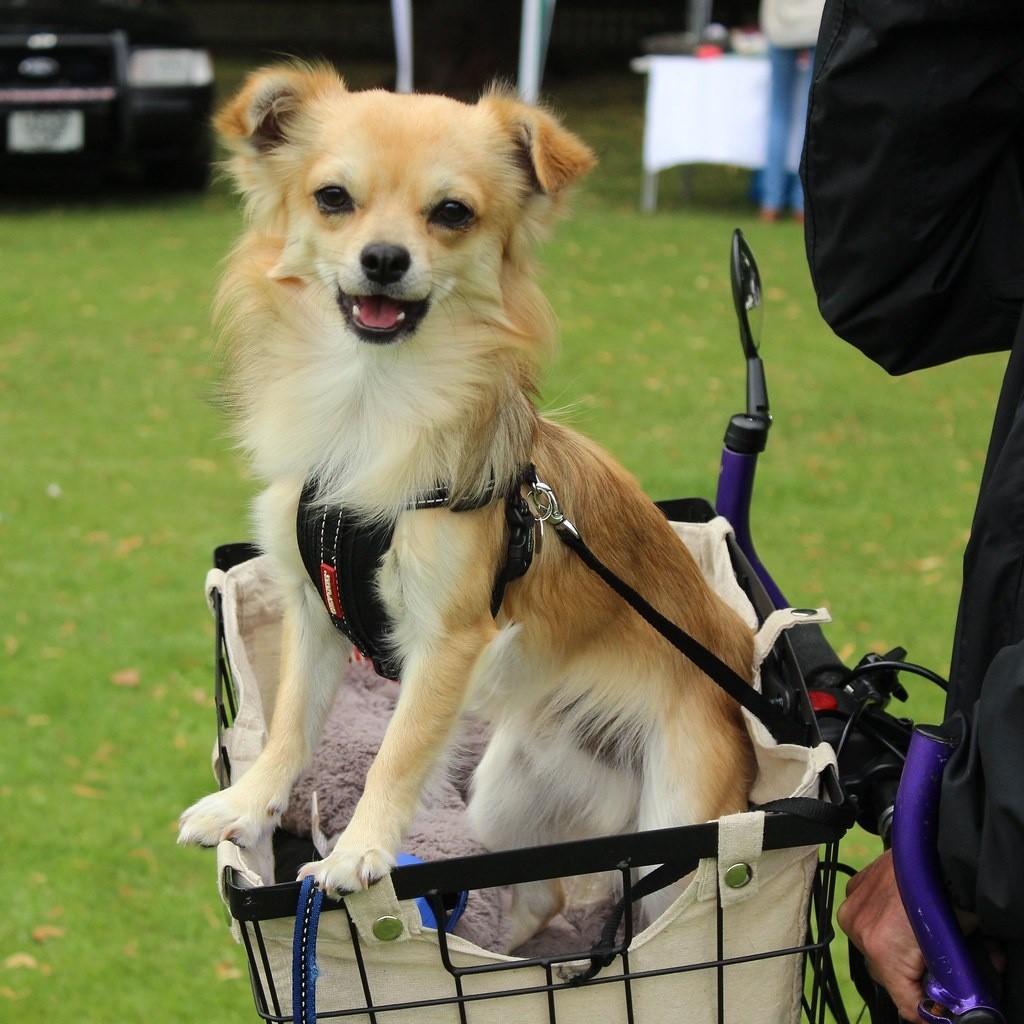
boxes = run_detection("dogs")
[173,59,759,898]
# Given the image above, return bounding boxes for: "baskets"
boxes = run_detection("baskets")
[208,493,864,1023]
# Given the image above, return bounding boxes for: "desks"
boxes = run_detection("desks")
[633,50,772,210]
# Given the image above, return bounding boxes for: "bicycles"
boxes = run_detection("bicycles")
[208,222,1011,1024]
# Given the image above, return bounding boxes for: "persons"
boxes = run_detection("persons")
[796,0,1024,1024]
[756,0,826,226]
